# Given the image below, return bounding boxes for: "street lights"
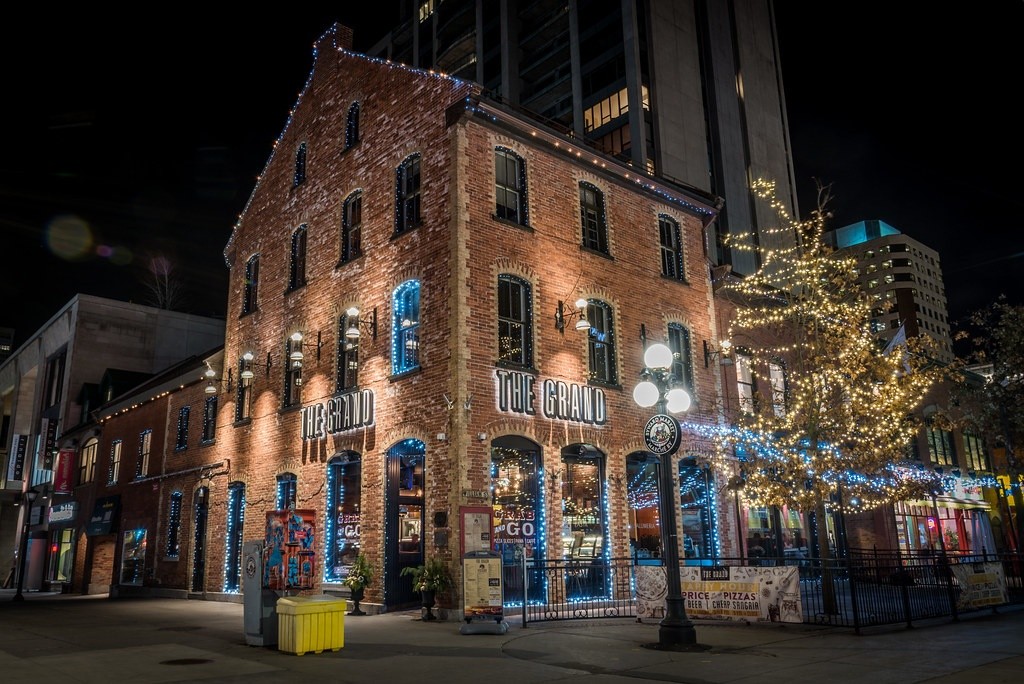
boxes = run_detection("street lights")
[630,341,698,648]
[12,488,41,601]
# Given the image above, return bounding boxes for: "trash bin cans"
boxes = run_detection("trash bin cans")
[276,593,348,656]
